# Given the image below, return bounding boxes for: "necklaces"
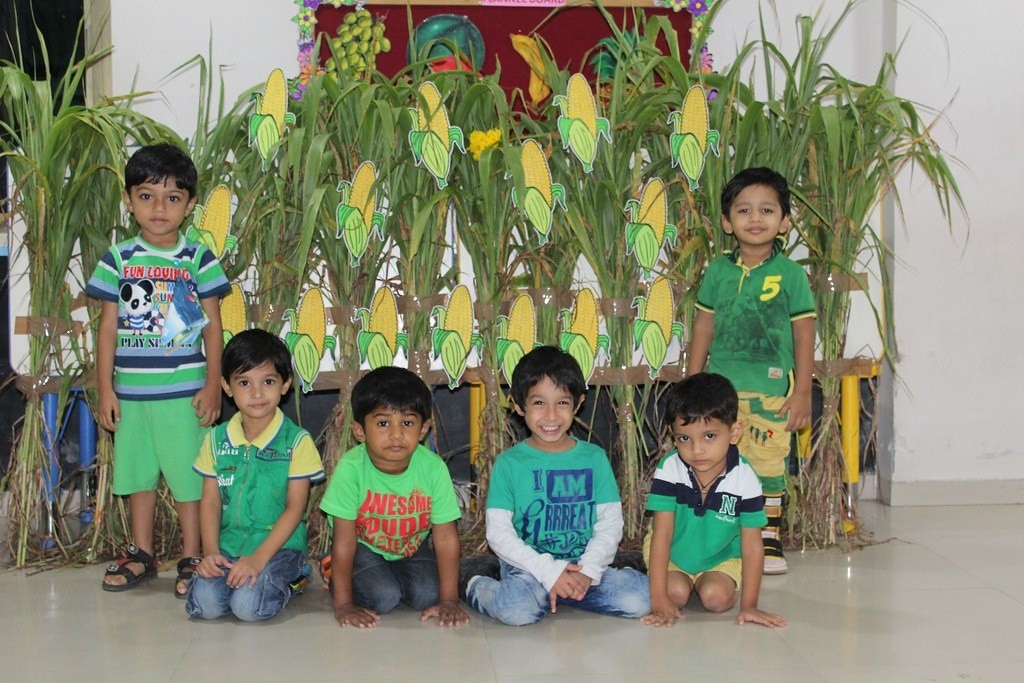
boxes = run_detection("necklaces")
[692,463,727,493]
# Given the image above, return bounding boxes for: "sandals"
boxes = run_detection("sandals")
[174,557,203,599]
[102,542,158,592]
[762,514,788,574]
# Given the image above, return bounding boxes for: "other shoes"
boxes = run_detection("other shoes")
[609,550,647,574]
[459,553,501,598]
[318,551,335,590]
[289,563,312,599]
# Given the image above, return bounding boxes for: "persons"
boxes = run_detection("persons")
[185,328,327,623]
[84,142,234,599]
[684,166,818,575]
[457,345,652,626]
[320,366,470,629]
[640,373,788,627]
